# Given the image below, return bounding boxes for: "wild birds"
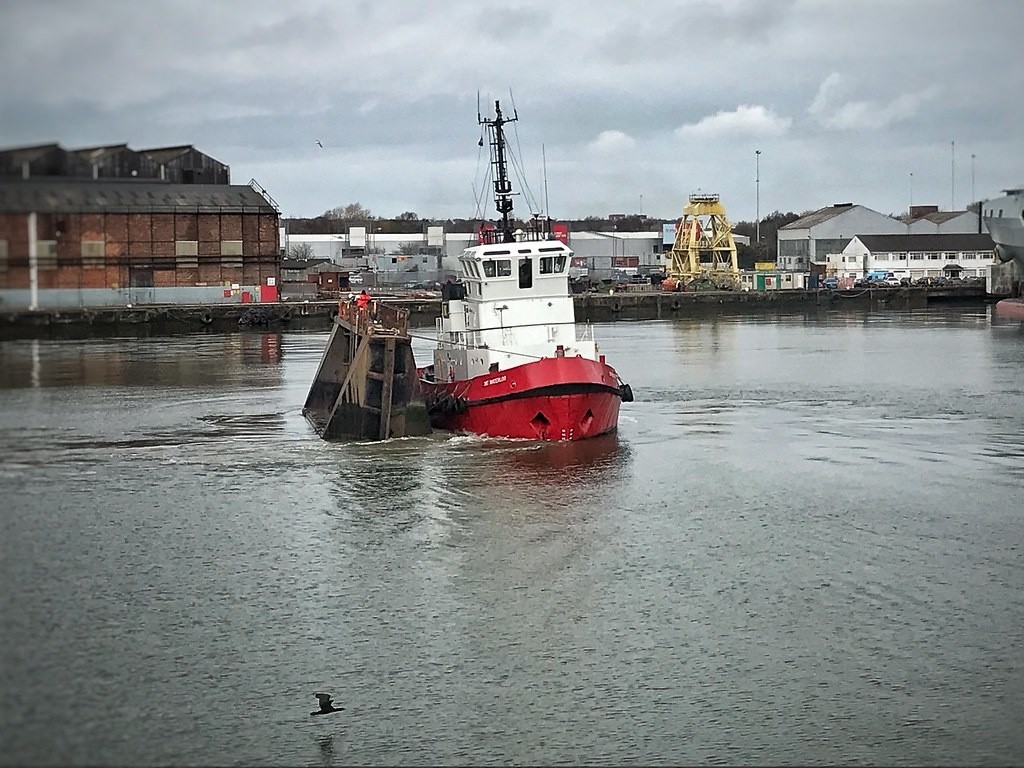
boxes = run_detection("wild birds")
[315,140,323,148]
[310,693,346,716]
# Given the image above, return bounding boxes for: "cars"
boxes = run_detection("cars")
[961,275,985,285]
[948,277,961,286]
[916,276,933,287]
[884,276,901,288]
[900,277,916,288]
[929,276,949,288]
[822,277,839,290]
[853,277,888,289]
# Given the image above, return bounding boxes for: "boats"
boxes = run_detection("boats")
[415,86,635,446]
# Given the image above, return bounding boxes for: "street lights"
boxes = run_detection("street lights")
[951,141,955,212]
[755,150,762,240]
[910,173,913,206]
[971,154,975,204]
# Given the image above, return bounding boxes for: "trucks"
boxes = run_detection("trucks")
[863,270,894,283]
